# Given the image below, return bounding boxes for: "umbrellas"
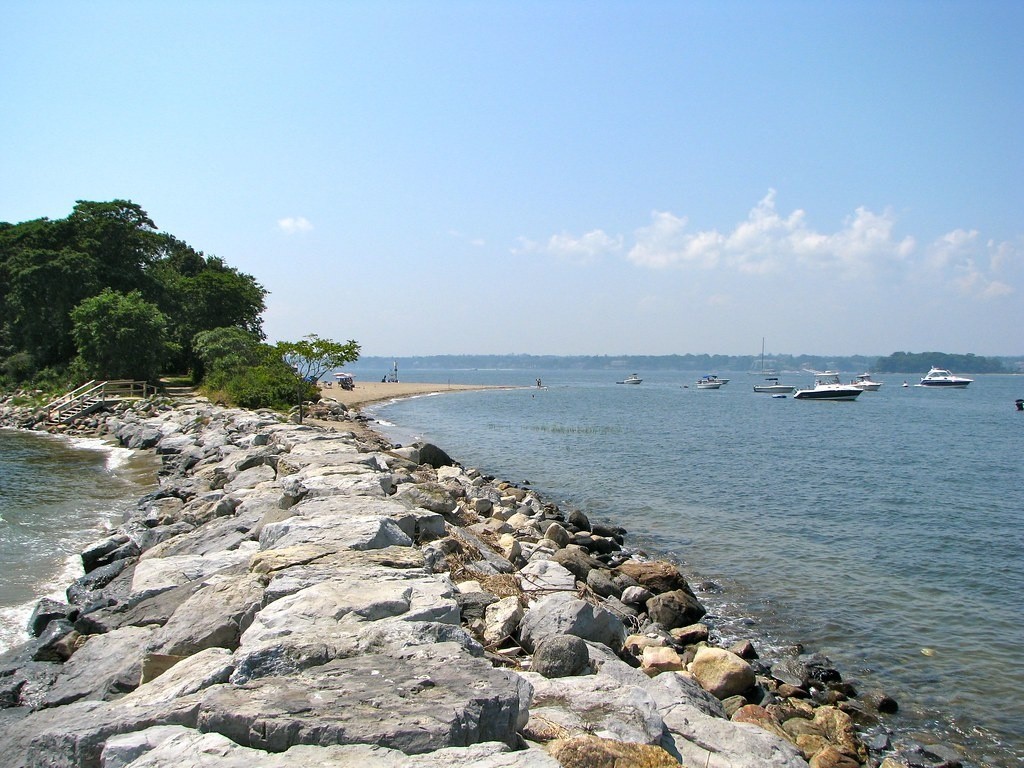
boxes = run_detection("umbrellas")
[333,373,356,378]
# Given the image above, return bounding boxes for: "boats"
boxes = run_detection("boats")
[616,372,642,385]
[771,394,788,398]
[754,377,795,392]
[902,380,908,387]
[793,371,864,401]
[700,374,730,384]
[843,372,884,390]
[695,379,722,389]
[920,364,975,388]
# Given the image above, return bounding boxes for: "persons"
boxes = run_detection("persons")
[536,378,541,386]
[341,378,355,391]
